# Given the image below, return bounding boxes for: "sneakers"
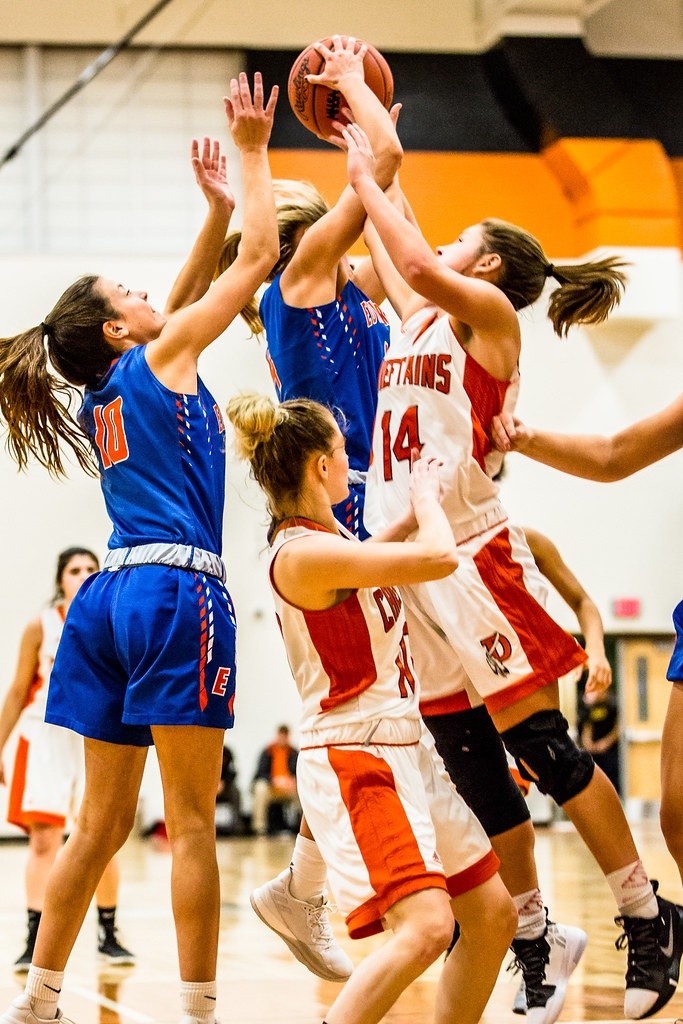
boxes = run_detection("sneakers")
[14,936,36,972]
[250,865,353,982]
[614,879,683,1020]
[1,991,78,1023]
[508,906,588,1024]
[96,927,136,966]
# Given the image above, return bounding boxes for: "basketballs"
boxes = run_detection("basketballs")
[286,35,391,142]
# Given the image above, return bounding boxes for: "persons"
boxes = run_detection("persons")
[0,548,138,974]
[582,692,619,795]
[250,726,302,842]
[492,461,614,694]
[1,69,281,1024]
[327,103,683,1023]
[216,747,245,837]
[661,601,683,882]
[217,34,405,986]
[222,394,521,1024]
